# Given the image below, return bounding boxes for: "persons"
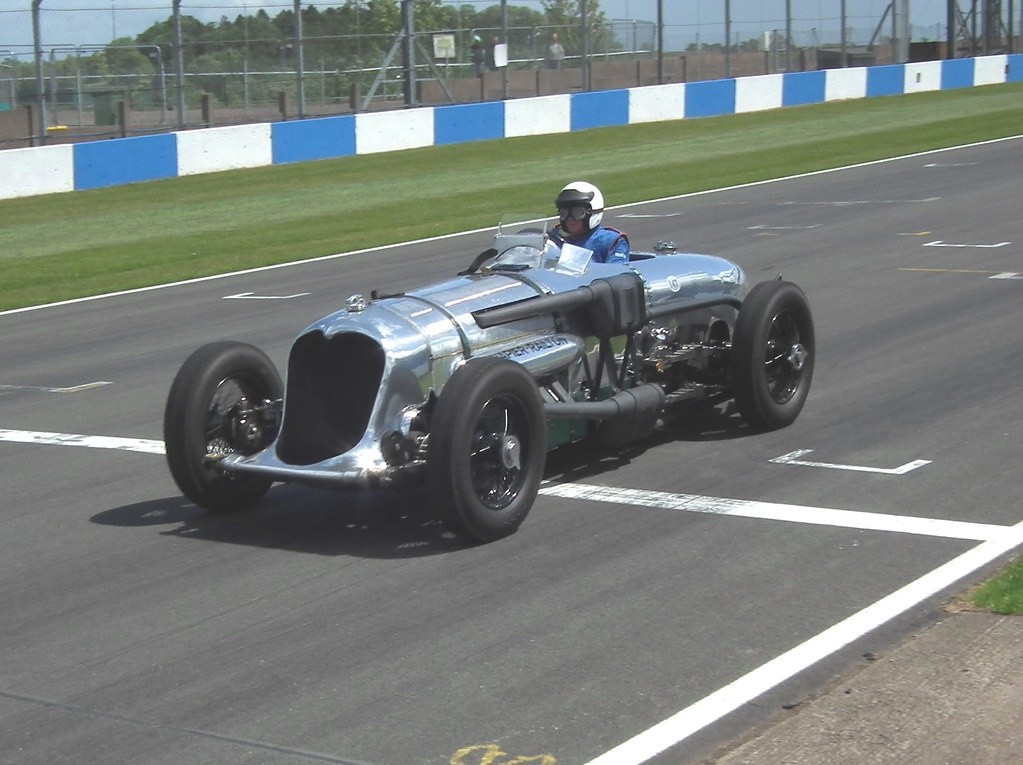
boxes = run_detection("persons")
[550,181,630,265]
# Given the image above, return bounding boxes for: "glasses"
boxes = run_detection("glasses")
[558,206,590,221]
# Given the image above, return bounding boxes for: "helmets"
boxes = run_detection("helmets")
[555,181,604,233]
